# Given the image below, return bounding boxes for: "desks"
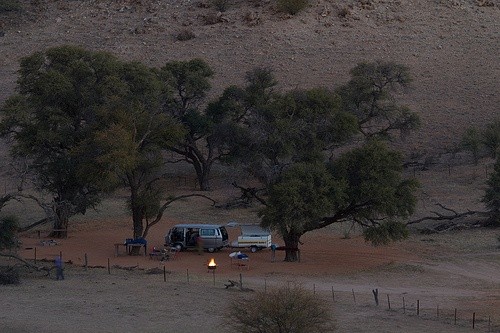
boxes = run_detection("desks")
[271,245,301,263]
[114,244,147,257]
[37,228,67,238]
[230,255,249,271]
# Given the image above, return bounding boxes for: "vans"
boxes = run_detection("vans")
[163,224,229,252]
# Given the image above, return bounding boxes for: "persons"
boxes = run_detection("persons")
[53,256,65,281]
